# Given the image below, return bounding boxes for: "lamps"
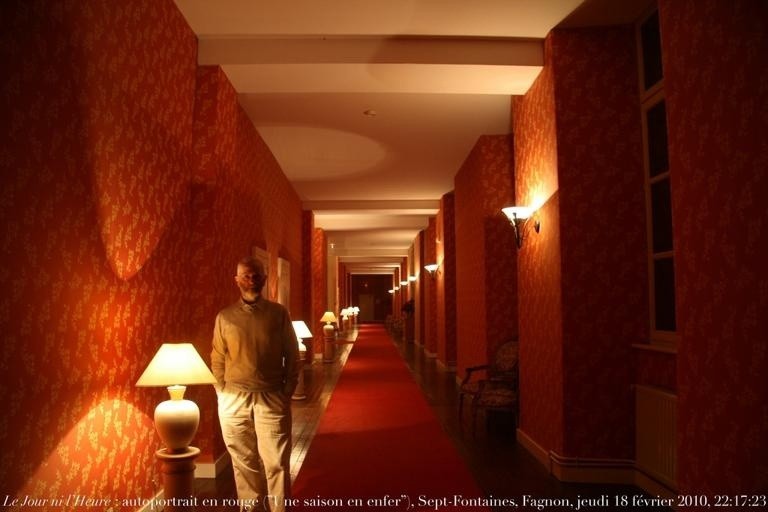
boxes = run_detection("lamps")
[502,205,538,248]
[134,342,217,455]
[291,305,361,352]
[387,262,438,296]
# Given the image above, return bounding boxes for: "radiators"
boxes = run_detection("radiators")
[634,385,677,493]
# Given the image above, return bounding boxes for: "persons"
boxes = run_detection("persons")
[211,256,304,512]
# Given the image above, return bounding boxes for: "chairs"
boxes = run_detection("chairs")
[457,337,519,447]
